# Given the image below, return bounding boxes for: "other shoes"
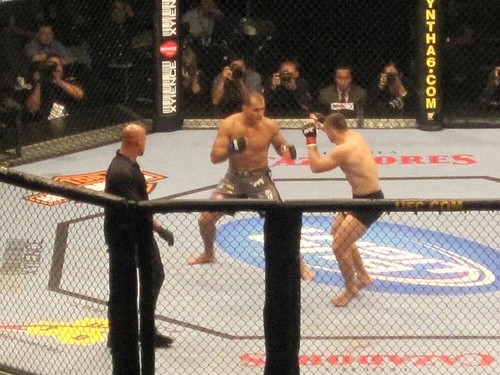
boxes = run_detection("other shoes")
[139,327,174,343]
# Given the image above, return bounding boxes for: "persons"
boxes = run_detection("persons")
[261,61,314,118]
[369,63,420,118]
[301,112,387,307]
[176,0,500,118]
[209,58,260,117]
[319,64,368,117]
[104,124,173,347]
[0,0,158,140]
[187,91,315,282]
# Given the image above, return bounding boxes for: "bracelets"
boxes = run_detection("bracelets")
[401,89,407,97]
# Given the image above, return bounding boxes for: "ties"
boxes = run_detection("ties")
[341,93,345,103]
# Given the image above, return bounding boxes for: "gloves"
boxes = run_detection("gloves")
[158,226,173,247]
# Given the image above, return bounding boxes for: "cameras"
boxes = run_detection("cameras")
[386,72,396,84]
[228,64,243,79]
[278,70,293,83]
[36,61,57,85]
